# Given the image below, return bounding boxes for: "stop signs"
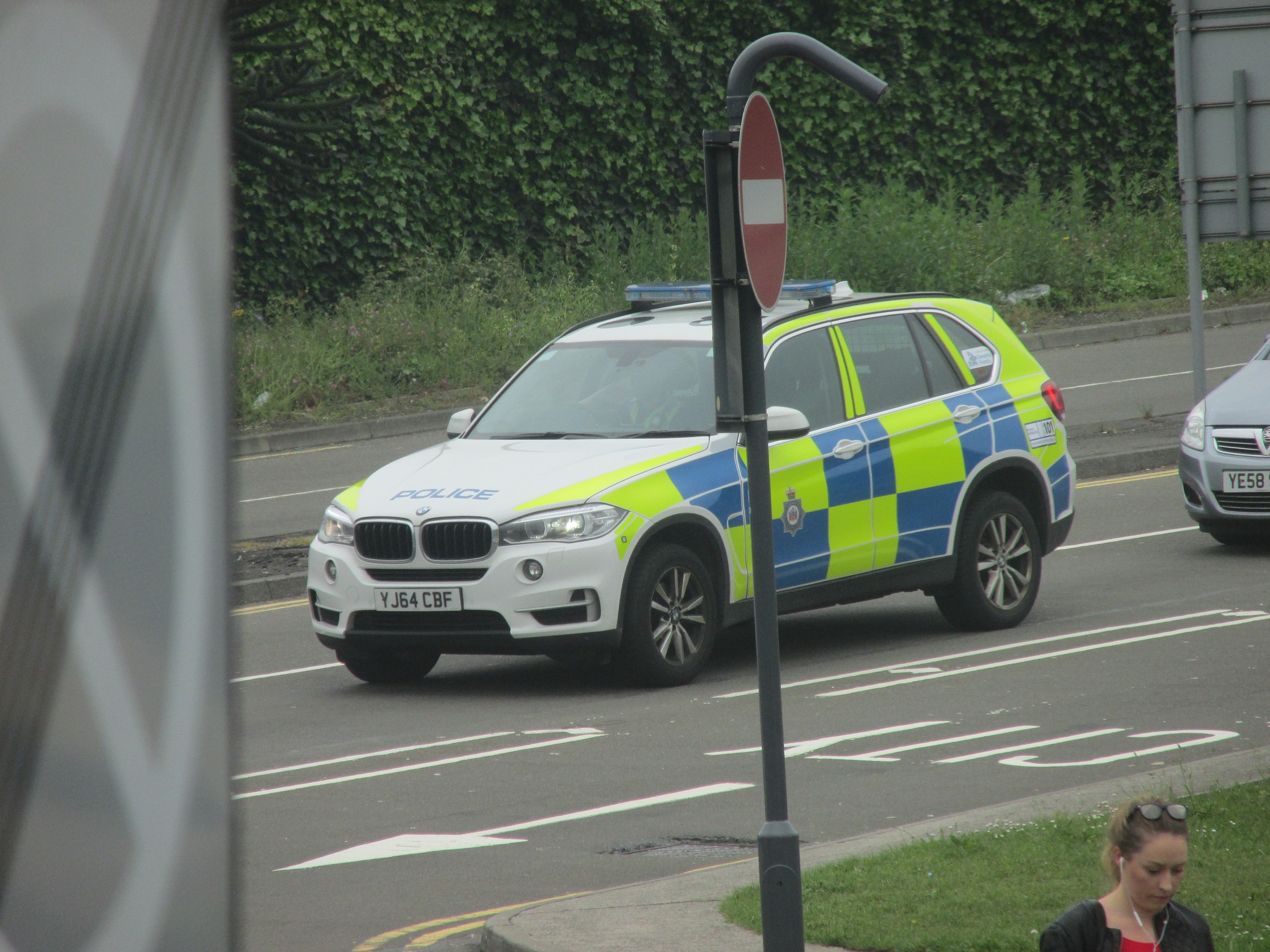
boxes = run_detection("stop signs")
[738,91,789,312]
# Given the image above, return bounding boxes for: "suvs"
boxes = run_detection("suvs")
[304,278,1078,687]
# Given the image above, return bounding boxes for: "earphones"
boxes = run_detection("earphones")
[1120,857,1125,875]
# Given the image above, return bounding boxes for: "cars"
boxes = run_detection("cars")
[1179,336,1270,548]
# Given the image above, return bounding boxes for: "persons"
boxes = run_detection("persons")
[1040,794,1216,952]
[617,357,686,434]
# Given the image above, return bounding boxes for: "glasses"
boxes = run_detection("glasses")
[1126,802,1189,823]
